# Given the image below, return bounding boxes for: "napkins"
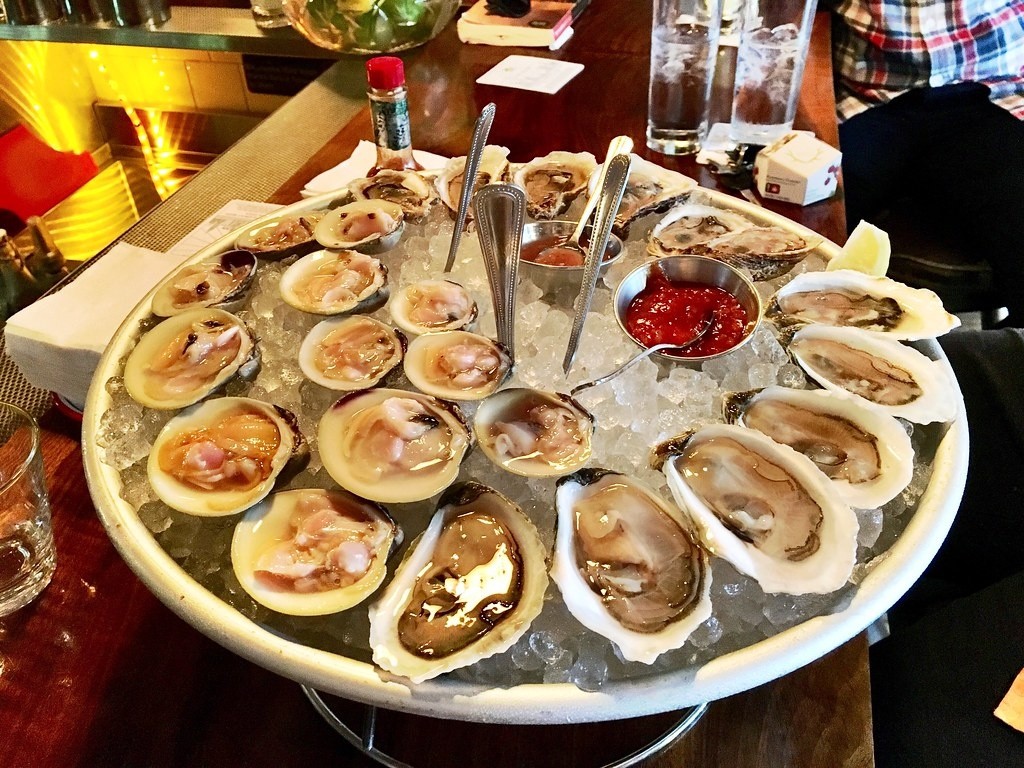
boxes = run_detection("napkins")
[476,55,586,95]
[4,240,188,406]
[696,118,794,166]
[299,138,449,198]
[677,14,764,48]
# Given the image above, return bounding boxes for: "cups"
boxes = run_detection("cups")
[250,0,293,30]
[646,0,725,155]
[702,0,759,46]
[729,0,818,146]
[0,402,57,616]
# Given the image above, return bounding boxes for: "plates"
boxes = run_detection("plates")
[81,168,970,723]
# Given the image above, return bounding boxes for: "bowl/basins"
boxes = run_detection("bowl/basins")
[520,220,625,271]
[614,255,763,361]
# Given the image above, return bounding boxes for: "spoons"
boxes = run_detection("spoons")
[533,136,634,265]
[571,311,715,398]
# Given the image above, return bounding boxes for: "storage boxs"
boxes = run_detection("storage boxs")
[752,130,842,207]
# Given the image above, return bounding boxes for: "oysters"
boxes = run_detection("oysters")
[125,143,963,685]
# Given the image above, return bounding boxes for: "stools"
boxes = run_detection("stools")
[871,199,993,297]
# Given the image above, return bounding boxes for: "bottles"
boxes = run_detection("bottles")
[366,57,426,178]
